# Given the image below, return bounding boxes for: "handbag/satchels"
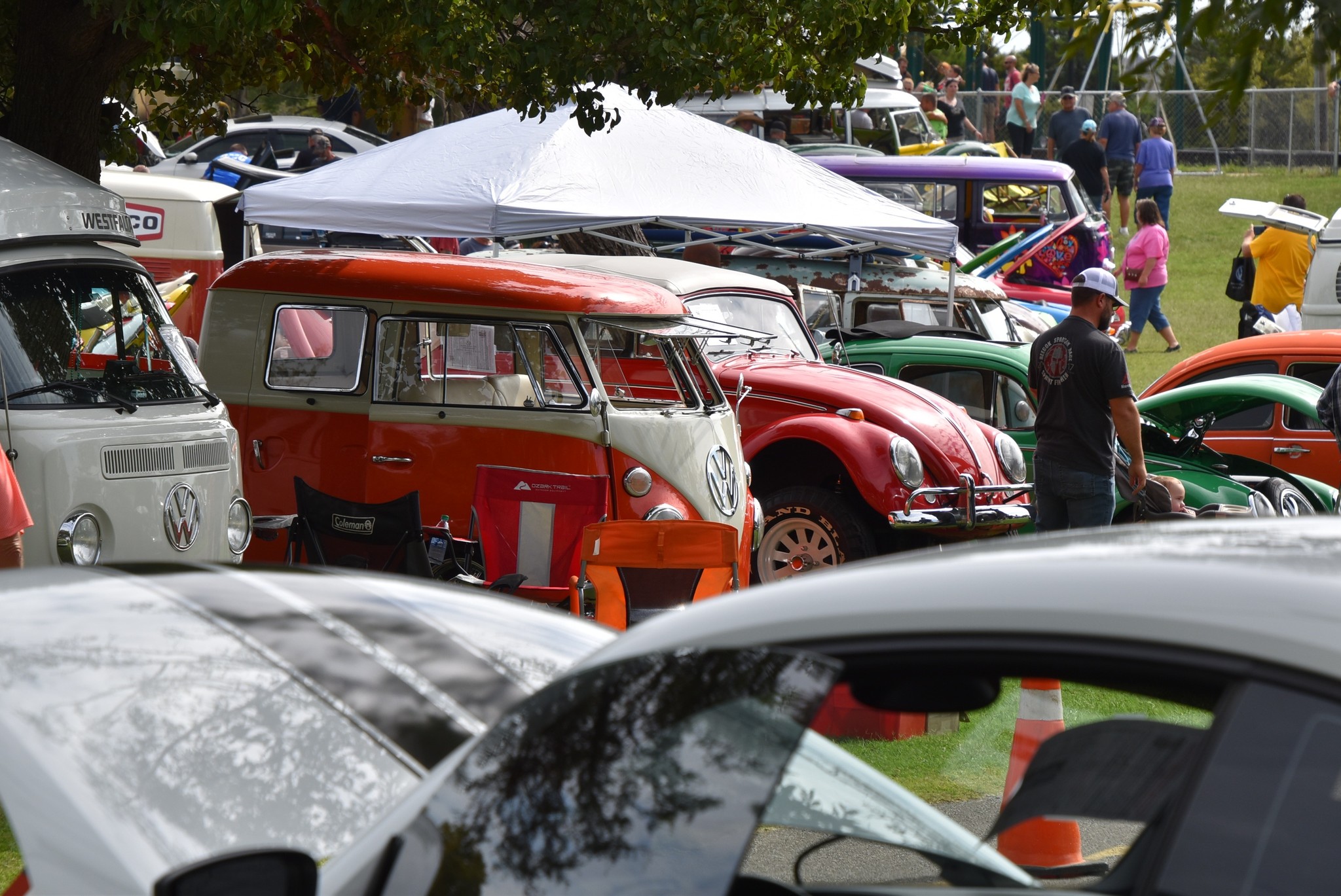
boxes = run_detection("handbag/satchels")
[1124,267,1143,282]
[1225,247,1257,302]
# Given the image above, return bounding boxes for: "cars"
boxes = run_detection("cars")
[101,96,396,192]
[784,140,1001,221]
[68,160,440,380]
[467,247,1045,587]
[1138,329,1341,493]
[113,61,224,144]
[632,201,1133,359]
[816,319,1341,535]
[0,516,1341,896]
[1218,197,1341,330]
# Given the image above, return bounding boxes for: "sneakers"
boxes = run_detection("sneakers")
[1124,348,1137,353]
[1164,344,1181,353]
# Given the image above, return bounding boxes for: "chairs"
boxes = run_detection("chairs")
[482,373,546,408]
[422,465,610,603]
[251,475,528,594]
[959,140,1058,214]
[399,378,502,407]
[570,520,740,631]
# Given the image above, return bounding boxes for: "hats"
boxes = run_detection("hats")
[1100,91,1126,103]
[1072,267,1129,308]
[1081,119,1097,134]
[1150,117,1164,127]
[1061,86,1075,99]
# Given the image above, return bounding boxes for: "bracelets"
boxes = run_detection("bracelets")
[973,130,977,136]
[1024,118,1029,124]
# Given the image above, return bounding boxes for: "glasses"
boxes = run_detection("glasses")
[1112,301,1120,311]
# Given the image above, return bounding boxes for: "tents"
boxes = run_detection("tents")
[237,79,960,400]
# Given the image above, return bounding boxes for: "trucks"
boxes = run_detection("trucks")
[0,137,255,574]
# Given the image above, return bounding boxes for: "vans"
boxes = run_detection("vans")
[196,250,768,636]
[674,52,945,163]
[795,156,1116,297]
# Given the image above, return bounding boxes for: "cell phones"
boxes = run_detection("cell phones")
[1253,225,1267,235]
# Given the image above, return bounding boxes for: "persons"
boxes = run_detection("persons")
[1048,86,1092,212]
[289,128,325,173]
[1027,267,1147,533]
[1151,474,1186,513]
[840,51,1023,155]
[312,135,343,170]
[1237,193,1317,340]
[768,121,790,147]
[200,143,253,188]
[1133,117,1176,232]
[1061,119,1111,212]
[1112,198,1181,354]
[316,84,360,127]
[1098,93,1143,236]
[732,110,754,134]
[1005,63,1042,159]
